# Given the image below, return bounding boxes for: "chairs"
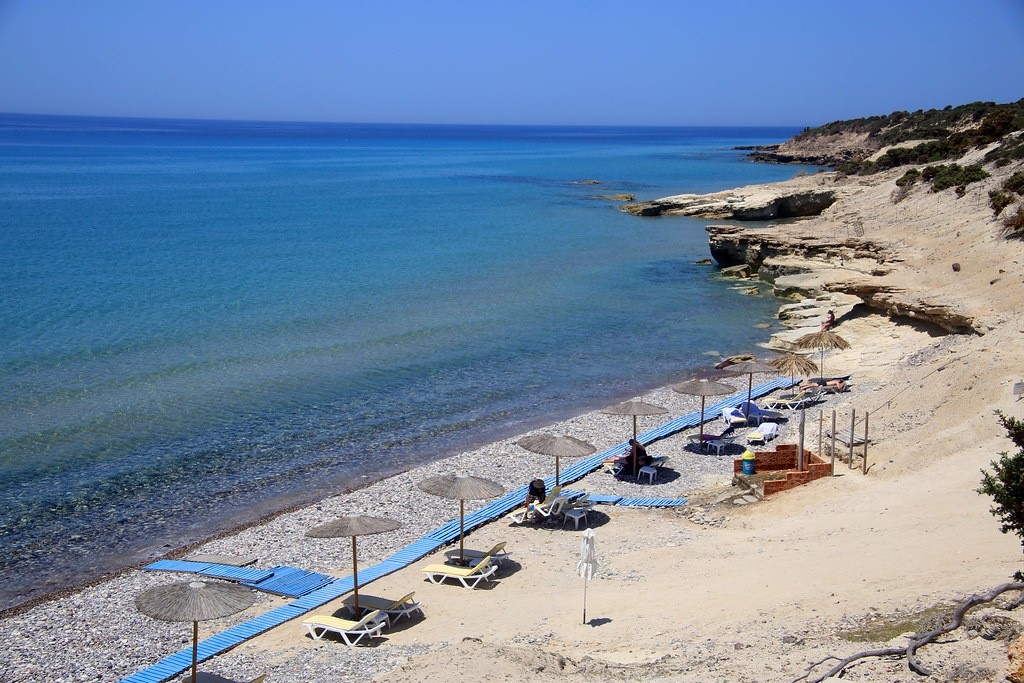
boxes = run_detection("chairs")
[602,452,669,476]
[720,407,748,429]
[558,491,591,516]
[741,399,785,423]
[420,555,498,590]
[443,539,515,567]
[746,420,779,446]
[340,590,423,624]
[507,484,564,524]
[686,425,736,446]
[780,382,824,406]
[762,389,806,411]
[799,375,855,392]
[808,371,856,384]
[301,608,387,650]
[180,670,270,683]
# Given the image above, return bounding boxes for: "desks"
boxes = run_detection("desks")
[637,466,660,484]
[362,611,391,636]
[706,439,727,455]
[564,509,587,529]
[748,412,763,426]
[469,557,493,568]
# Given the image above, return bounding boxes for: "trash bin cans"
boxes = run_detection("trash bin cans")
[742,451,755,477]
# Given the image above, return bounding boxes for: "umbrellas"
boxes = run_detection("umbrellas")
[135,580,257,683]
[723,360,779,416]
[575,528,599,624]
[793,329,851,380]
[304,514,401,619]
[417,471,504,565]
[516,433,597,487]
[770,353,817,395]
[671,378,737,446]
[600,400,668,475]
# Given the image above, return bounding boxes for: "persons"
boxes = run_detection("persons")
[820,310,835,331]
[799,379,846,392]
[614,439,647,465]
[525,478,546,511]
